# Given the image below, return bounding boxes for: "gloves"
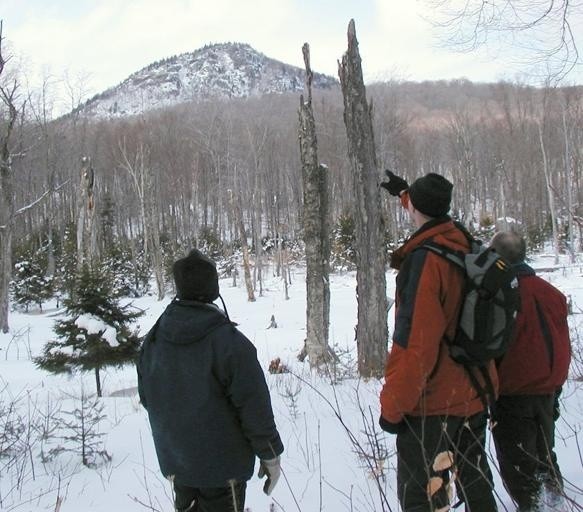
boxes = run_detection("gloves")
[258,455,281,496]
[379,415,407,434]
[380,169,410,198]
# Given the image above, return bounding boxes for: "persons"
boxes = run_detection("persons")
[372,165,503,511]
[480,234,571,512]
[136,249,283,512]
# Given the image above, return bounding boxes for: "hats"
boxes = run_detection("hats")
[408,173,454,219]
[172,248,219,304]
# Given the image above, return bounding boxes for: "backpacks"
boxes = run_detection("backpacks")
[411,220,522,367]
